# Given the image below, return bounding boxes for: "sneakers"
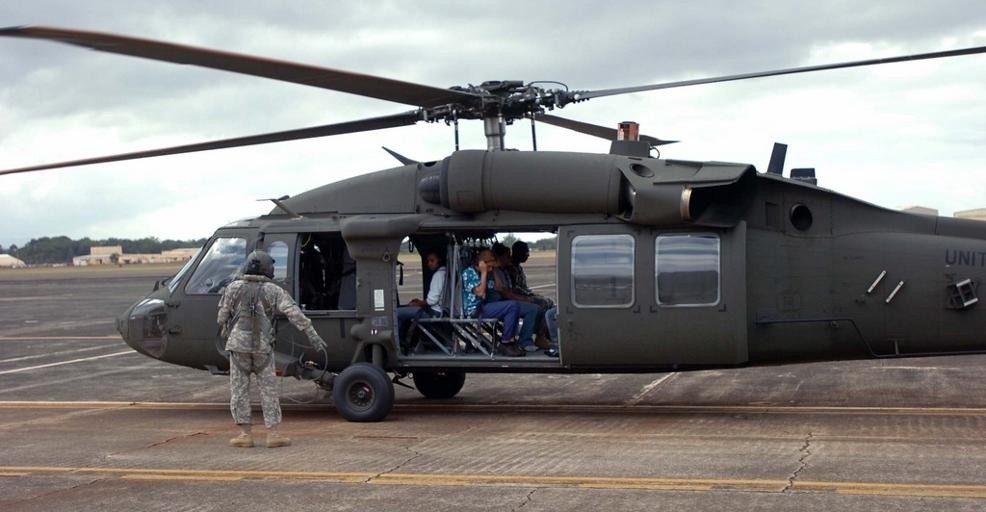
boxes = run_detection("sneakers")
[501,336,552,356]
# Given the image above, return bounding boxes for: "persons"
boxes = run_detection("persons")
[217,249,319,448]
[397,241,559,356]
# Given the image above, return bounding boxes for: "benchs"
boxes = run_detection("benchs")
[409,243,509,358]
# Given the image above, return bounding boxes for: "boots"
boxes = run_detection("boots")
[266,426,291,447]
[230,425,254,447]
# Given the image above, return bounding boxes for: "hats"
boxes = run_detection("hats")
[476,250,495,269]
[493,241,509,259]
[512,240,529,255]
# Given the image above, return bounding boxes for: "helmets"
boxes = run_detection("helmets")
[245,249,274,278]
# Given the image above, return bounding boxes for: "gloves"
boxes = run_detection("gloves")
[310,332,328,352]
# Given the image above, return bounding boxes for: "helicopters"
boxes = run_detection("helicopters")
[0,24,986,423]
